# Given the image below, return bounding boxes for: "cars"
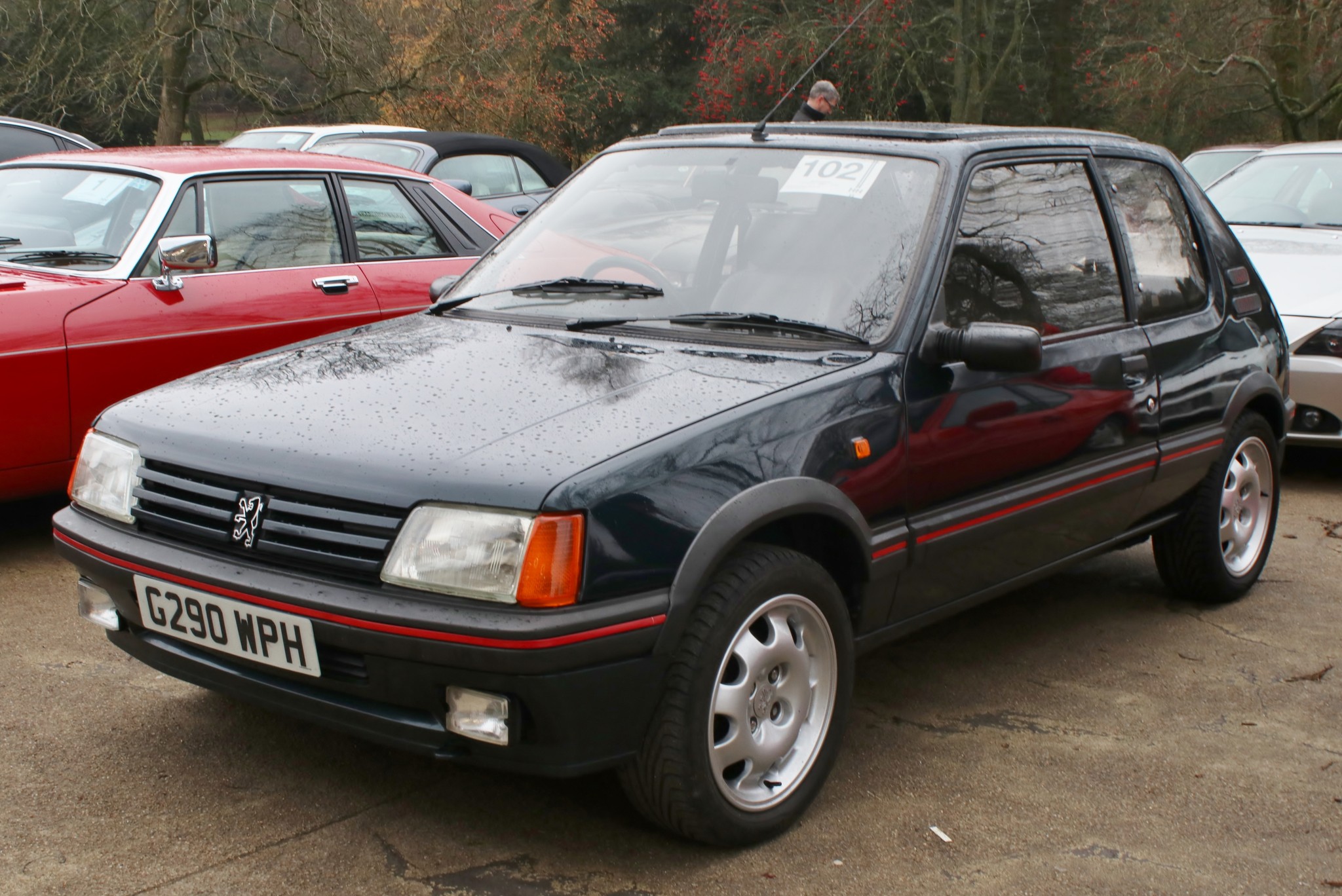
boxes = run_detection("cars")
[0,146,678,500]
[0,116,104,164]
[1134,141,1342,450]
[302,131,574,219]
[218,124,428,153]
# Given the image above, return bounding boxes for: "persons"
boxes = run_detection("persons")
[792,80,839,121]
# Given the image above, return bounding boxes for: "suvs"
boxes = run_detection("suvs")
[53,124,1298,851]
